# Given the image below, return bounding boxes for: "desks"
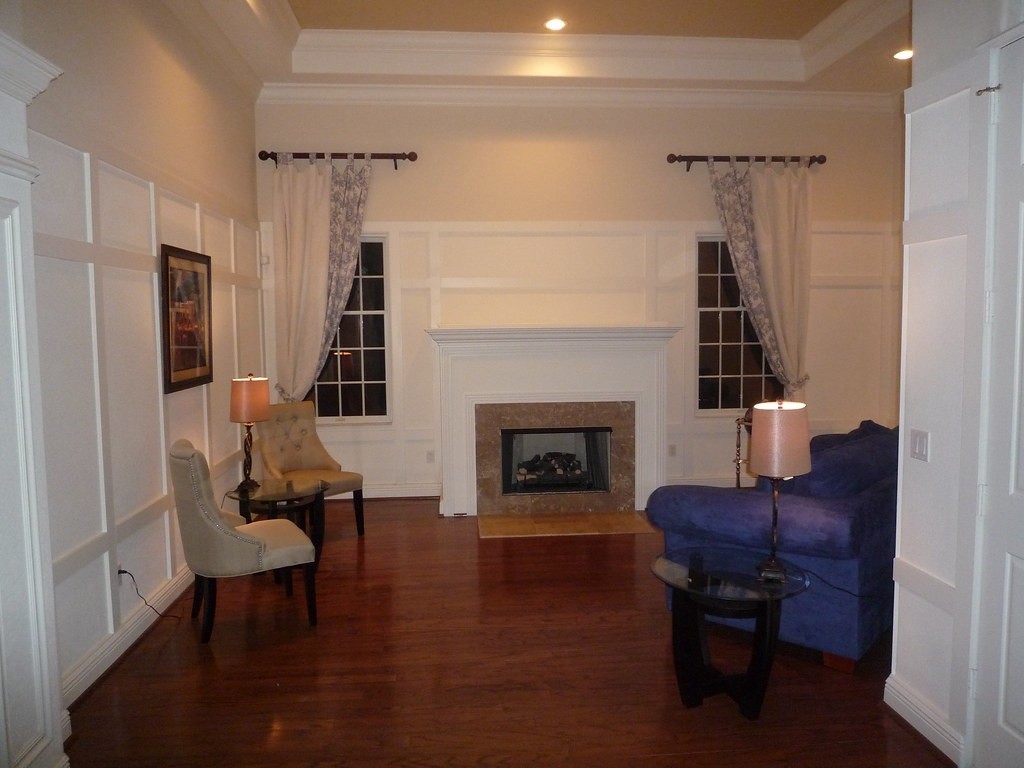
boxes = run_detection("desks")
[649,546,811,722]
[227,479,330,598]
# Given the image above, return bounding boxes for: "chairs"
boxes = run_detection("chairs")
[169,439,317,644]
[257,401,365,536]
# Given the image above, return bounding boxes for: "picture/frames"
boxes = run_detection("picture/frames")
[160,244,213,395]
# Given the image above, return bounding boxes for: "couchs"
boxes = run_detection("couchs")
[646,419,899,676]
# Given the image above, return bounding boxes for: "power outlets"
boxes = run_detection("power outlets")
[118,563,123,585]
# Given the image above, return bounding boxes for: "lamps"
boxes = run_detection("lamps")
[750,399,812,580]
[230,373,271,491]
